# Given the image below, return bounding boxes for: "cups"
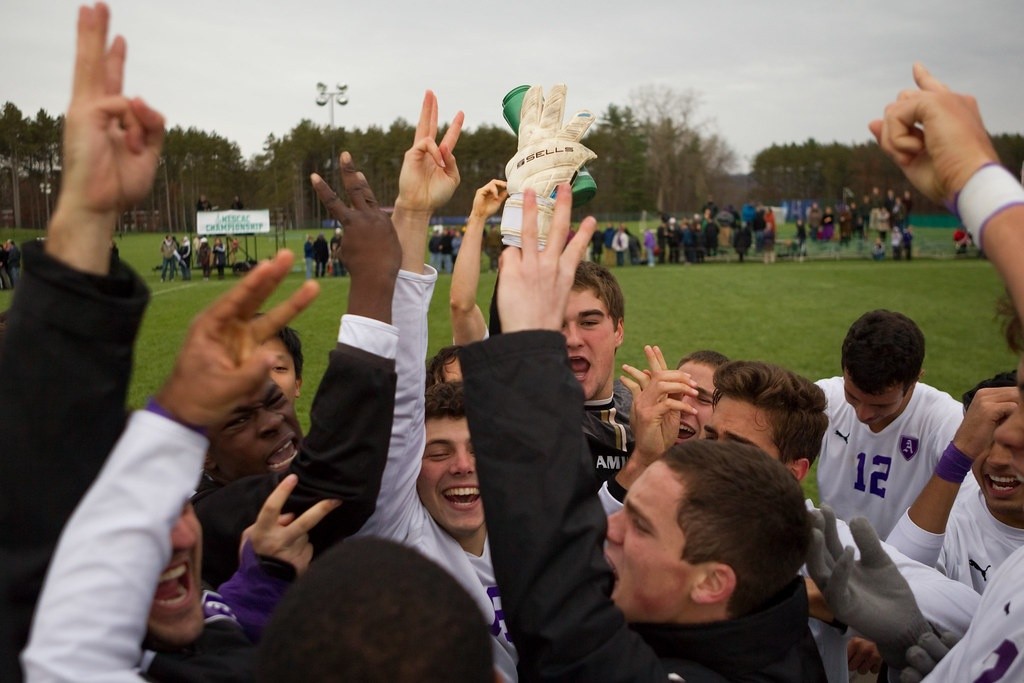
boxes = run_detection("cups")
[502,83,598,209]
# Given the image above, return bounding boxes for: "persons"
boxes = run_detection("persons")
[1,0,1024,683]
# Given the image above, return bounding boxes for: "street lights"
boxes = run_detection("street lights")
[315,80,348,227]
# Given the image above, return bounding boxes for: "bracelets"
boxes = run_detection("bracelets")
[950,162,1024,250]
[935,441,974,483]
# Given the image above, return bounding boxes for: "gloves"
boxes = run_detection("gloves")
[901,631,962,683]
[499,82,599,247]
[805,501,935,667]
